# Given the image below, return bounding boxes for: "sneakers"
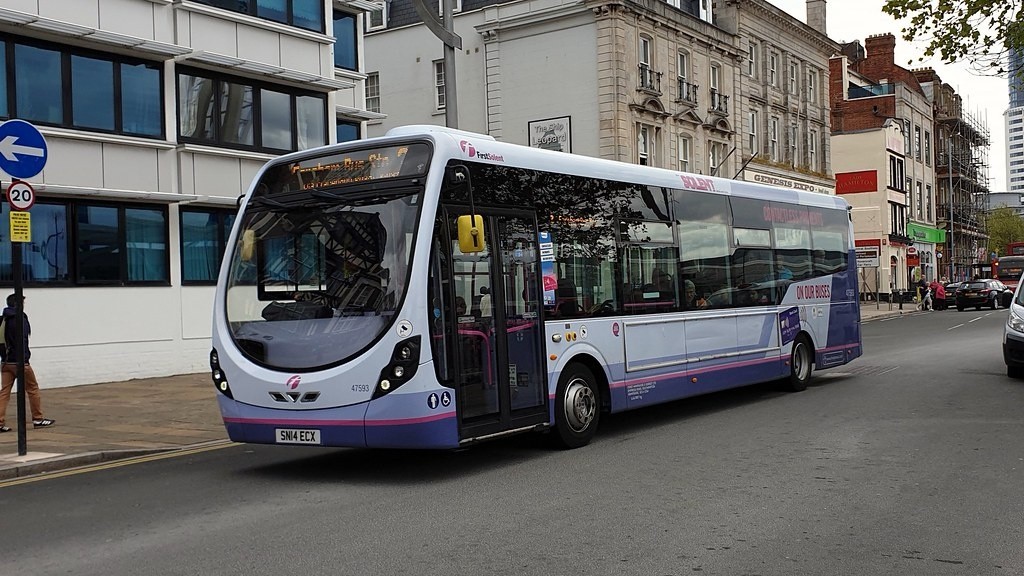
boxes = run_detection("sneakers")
[34,418,55,429]
[0,426,12,432]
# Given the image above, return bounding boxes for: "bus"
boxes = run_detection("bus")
[992,256,1024,293]
[207,124,864,456]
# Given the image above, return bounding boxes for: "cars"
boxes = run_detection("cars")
[955,278,1013,312]
[1001,270,1024,379]
[943,282,963,309]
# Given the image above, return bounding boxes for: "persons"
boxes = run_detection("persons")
[452,280,507,332]
[638,248,832,314]
[295,248,379,312]
[0,292,56,432]
[915,271,950,313]
